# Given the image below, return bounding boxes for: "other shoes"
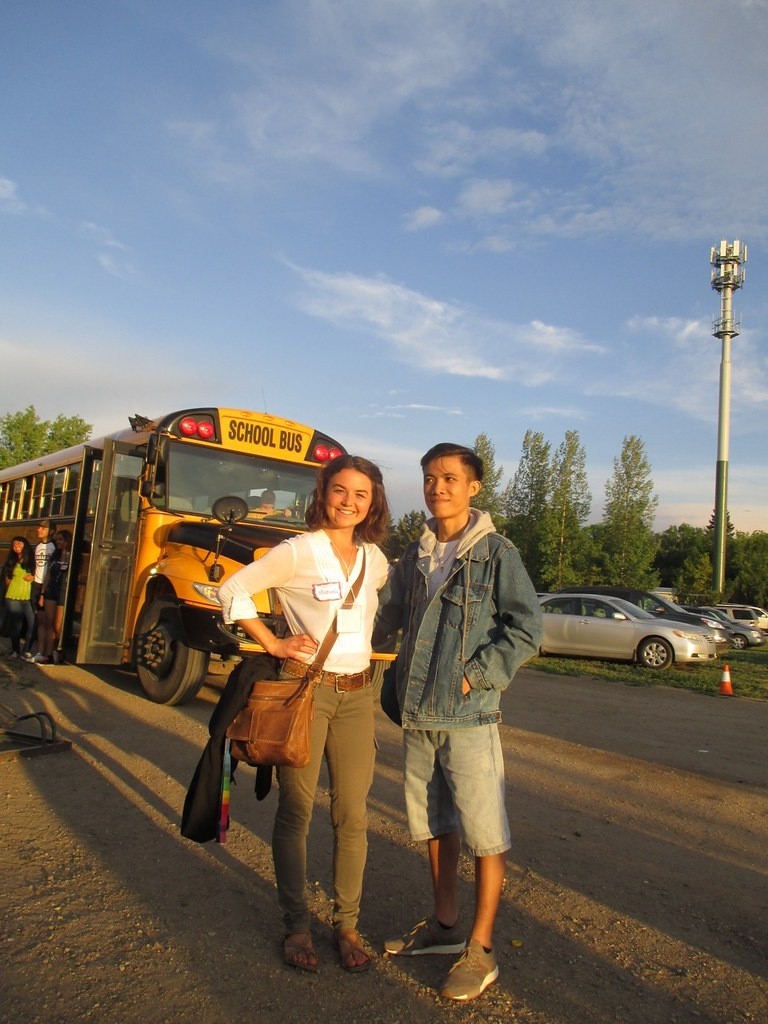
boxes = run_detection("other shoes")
[20,651,35,663]
[8,651,20,660]
[39,655,53,664]
[34,653,43,659]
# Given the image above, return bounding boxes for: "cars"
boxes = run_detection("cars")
[709,603,768,636]
[678,606,766,651]
[536,592,717,671]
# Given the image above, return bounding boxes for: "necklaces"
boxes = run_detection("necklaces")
[433,544,458,572]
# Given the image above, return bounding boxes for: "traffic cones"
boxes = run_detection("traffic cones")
[715,661,736,697]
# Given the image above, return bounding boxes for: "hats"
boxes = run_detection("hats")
[37,519,57,529]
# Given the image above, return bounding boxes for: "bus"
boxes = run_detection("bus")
[0,407,357,707]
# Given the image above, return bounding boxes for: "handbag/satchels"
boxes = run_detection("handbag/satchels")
[380,656,402,727]
[226,680,315,768]
[0,611,12,638]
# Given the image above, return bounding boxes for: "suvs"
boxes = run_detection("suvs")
[554,585,735,651]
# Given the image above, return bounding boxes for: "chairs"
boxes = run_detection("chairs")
[592,608,606,617]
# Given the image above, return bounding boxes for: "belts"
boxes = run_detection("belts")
[283,659,372,694]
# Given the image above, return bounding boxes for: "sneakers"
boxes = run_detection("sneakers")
[383,914,467,954]
[441,938,499,1001]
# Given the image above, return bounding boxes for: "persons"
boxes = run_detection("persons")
[27,521,57,663]
[373,443,545,1001]
[218,455,393,973]
[37,530,84,666]
[247,490,292,519]
[0,536,35,663]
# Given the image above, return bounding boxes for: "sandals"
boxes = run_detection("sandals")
[279,928,319,971]
[332,929,370,974]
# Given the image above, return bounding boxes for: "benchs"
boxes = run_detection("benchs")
[541,607,571,614]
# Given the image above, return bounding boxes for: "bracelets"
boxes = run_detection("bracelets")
[40,593,44,595]
